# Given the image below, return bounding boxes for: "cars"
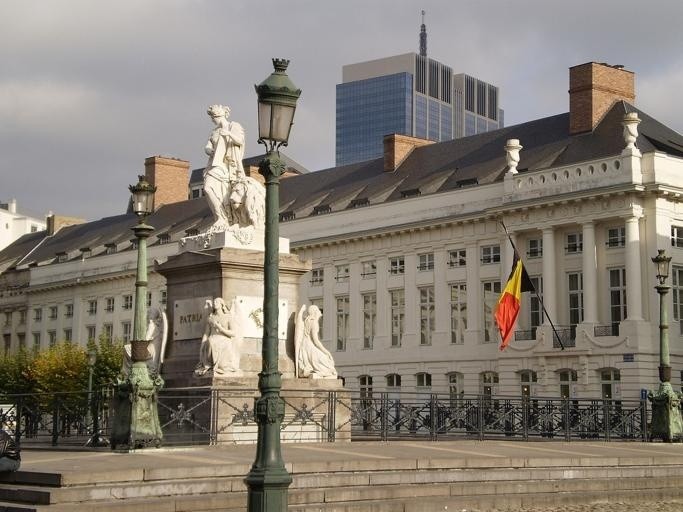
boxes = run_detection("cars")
[81,416,104,434]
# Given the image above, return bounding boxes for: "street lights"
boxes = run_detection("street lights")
[243,57,302,512]
[651,249,683,443]
[86,351,97,435]
[107,174,164,448]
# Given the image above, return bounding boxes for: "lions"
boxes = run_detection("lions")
[228,175,267,230]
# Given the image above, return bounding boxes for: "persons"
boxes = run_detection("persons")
[299,305,337,378]
[203,105,245,229]
[200,298,236,374]
[146,308,161,367]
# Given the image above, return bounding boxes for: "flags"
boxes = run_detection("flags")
[495,249,535,351]
